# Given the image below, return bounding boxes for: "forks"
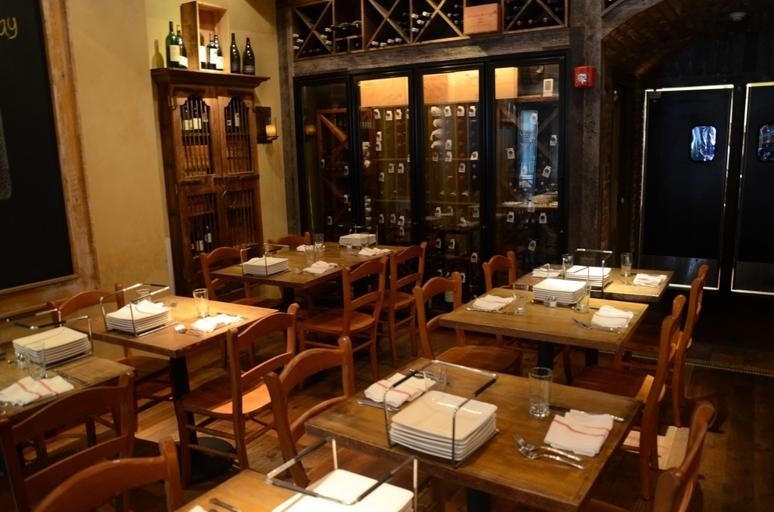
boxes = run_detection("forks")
[511,432,589,474]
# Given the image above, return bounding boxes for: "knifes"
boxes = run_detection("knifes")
[549,403,625,422]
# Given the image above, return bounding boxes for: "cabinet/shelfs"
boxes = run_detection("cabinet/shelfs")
[165,182,265,305]
[291,0,569,62]
[159,81,259,185]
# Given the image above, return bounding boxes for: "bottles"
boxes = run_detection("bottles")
[165,20,257,75]
[288,0,567,58]
[181,103,242,133]
[189,221,213,256]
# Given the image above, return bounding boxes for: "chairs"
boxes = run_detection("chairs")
[613,263,710,427]
[410,270,525,377]
[483,250,574,384]
[579,398,719,512]
[569,293,688,500]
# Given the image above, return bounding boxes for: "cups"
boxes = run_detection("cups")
[561,253,574,278]
[135,289,152,303]
[20,340,48,381]
[528,366,555,419]
[422,362,448,390]
[312,233,325,257]
[359,231,370,250]
[512,282,529,316]
[543,294,558,310]
[193,288,210,318]
[620,252,632,276]
[575,280,592,312]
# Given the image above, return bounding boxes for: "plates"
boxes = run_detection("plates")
[565,264,613,288]
[12,327,91,365]
[532,277,588,306]
[243,255,291,276]
[266,468,419,512]
[388,389,499,460]
[338,233,378,248]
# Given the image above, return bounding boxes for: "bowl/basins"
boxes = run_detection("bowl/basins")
[106,302,172,335]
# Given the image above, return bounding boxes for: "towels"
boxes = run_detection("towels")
[473,294,515,313]
[632,272,668,289]
[543,409,616,458]
[531,267,562,279]
[513,261,675,371]
[589,304,635,333]
[362,372,436,408]
